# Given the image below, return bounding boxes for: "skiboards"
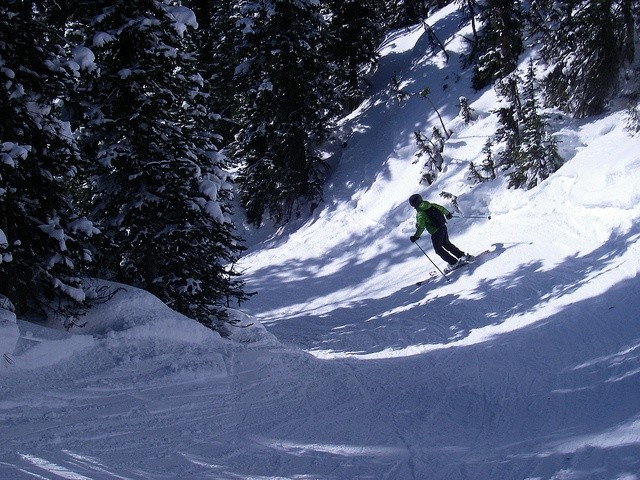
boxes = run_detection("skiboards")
[415,249,489,286]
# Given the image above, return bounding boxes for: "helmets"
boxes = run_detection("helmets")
[409,194,422,207]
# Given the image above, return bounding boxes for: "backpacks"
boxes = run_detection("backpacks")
[423,205,447,228]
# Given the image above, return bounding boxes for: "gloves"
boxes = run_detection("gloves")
[446,213,452,220]
[410,236,419,243]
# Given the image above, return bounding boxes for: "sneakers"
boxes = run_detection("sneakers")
[447,260,460,271]
[457,255,467,263]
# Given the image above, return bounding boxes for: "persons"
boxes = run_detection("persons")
[411,194,467,270]
